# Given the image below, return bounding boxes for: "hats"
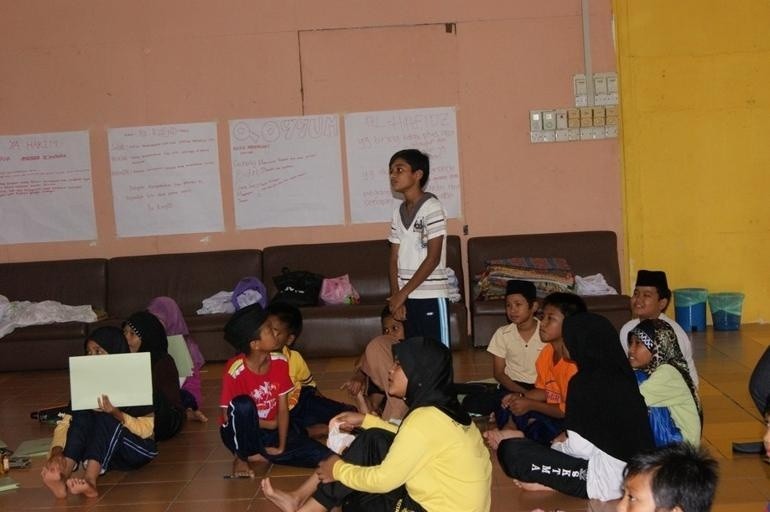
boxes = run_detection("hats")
[638,270,667,285]
[507,280,536,297]
[224,303,267,349]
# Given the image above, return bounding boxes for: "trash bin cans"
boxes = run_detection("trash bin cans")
[708,293,745,330]
[673,288,708,331]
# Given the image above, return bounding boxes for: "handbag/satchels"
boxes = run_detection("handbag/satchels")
[270,267,322,307]
[575,273,618,296]
[319,274,361,305]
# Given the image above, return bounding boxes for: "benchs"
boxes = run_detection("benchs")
[466,230,632,348]
[108,248,262,361]
[1,259,108,370]
[261,234,467,361]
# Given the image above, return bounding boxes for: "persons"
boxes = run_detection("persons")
[497,310,658,501]
[139,295,210,424]
[219,268,718,455]
[259,336,494,512]
[746,342,770,421]
[762,394,769,464]
[385,149,452,368]
[40,322,157,500]
[612,443,718,512]
[218,304,352,480]
[120,310,185,443]
